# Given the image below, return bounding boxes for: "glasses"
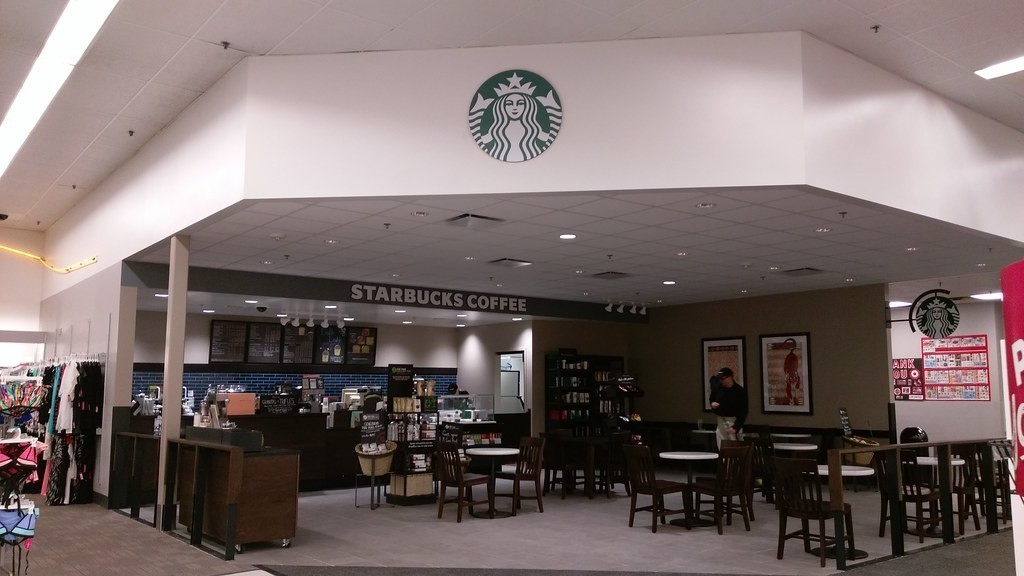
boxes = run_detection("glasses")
[719,375,730,381]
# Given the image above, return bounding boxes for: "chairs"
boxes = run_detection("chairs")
[495,437,546,516]
[624,429,1014,566]
[539,430,631,500]
[437,444,489,523]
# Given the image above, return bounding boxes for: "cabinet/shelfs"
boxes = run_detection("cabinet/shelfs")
[544,353,624,427]
[386,412,438,506]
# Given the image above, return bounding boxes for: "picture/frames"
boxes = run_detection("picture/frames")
[758,332,813,416]
[700,336,748,412]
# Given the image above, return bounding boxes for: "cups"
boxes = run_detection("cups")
[334,345,342,356]
[322,351,329,362]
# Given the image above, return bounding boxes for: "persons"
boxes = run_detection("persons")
[446,383,473,410]
[709,367,750,478]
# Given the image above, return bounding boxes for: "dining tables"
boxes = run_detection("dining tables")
[767,440,818,450]
[772,432,814,438]
[694,430,716,434]
[801,465,875,476]
[903,455,968,466]
[658,451,721,459]
[440,418,520,519]
[561,437,613,493]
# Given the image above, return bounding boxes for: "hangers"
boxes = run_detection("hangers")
[0,352,99,511]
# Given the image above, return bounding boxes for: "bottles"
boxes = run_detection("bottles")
[600,393,625,415]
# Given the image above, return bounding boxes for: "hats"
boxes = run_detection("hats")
[716,368,731,378]
